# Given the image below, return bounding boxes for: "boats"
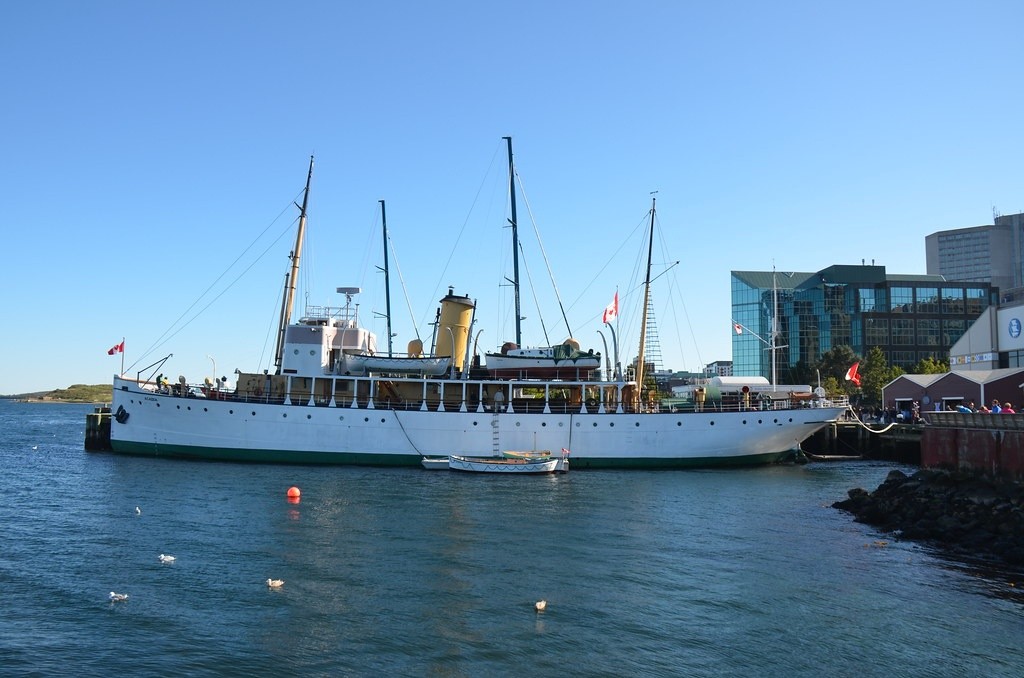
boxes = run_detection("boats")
[421,448,571,475]
[108,137,851,469]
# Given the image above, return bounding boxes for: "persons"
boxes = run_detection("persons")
[967,402,977,412]
[955,405,972,413]
[156,374,162,388]
[854,406,913,425]
[1000,403,1015,414]
[162,377,169,386]
[979,405,989,413]
[990,399,1002,414]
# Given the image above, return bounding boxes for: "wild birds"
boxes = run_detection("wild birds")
[265,579,286,587]
[109,591,129,602]
[535,600,546,609]
[134,506,140,514]
[157,554,176,562]
[32,446,38,450]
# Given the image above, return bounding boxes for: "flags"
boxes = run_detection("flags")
[845,363,860,386]
[108,341,124,355]
[733,322,743,335]
[603,292,618,323]
